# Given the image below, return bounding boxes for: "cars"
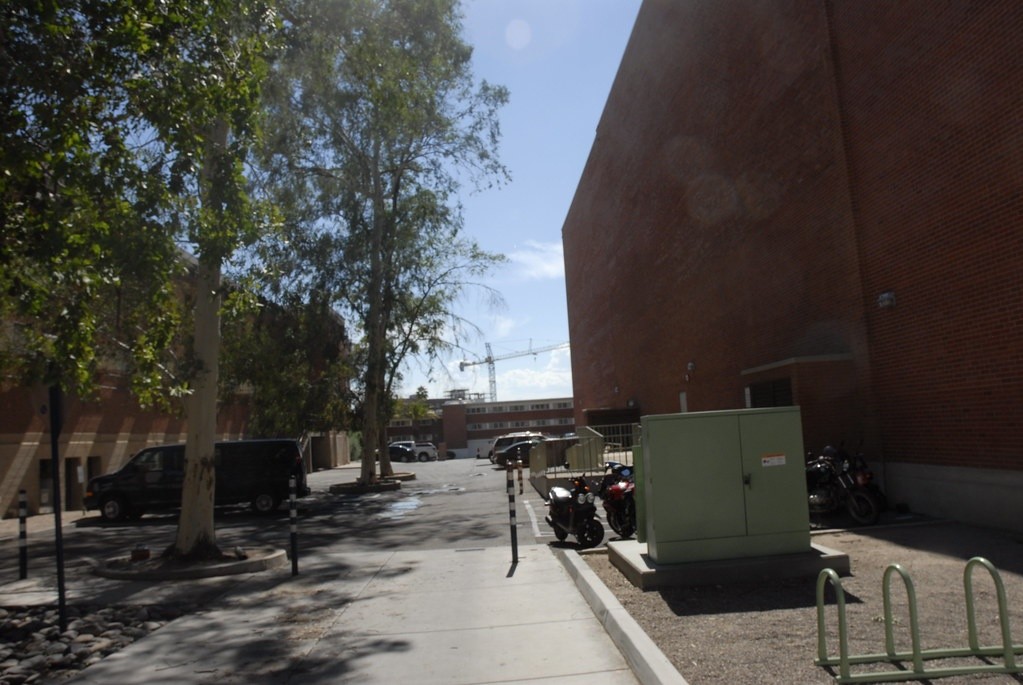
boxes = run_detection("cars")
[375,445,415,462]
[494,439,544,468]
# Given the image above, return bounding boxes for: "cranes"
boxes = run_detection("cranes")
[459,336,572,400]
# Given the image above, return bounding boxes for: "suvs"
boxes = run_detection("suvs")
[489,433,550,464]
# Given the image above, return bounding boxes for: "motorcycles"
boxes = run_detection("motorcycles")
[803,439,889,528]
[544,461,605,547]
[598,463,639,540]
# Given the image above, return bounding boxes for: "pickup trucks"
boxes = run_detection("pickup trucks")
[389,440,438,462]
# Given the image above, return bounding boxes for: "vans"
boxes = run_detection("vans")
[84,439,310,518]
